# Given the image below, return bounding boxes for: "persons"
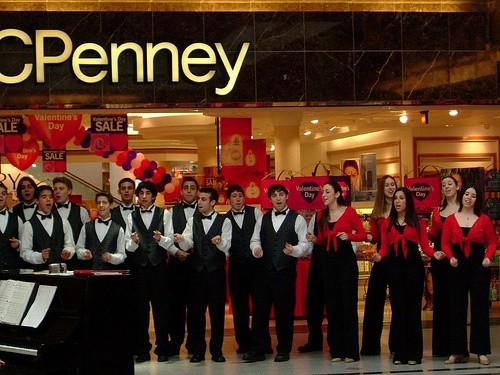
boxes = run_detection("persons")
[20,185,75,270]
[440,182,497,365]
[428,176,462,358]
[343,160,359,192]
[242,183,309,362]
[53,176,91,246]
[173,187,232,363]
[110,177,137,233]
[154,176,200,356]
[9,176,38,223]
[360,174,398,358]
[423,268,433,310]
[224,184,274,354]
[75,192,127,271]
[364,187,445,365]
[306,180,367,363]
[297,205,358,353]
[0,183,23,271]
[125,179,174,362]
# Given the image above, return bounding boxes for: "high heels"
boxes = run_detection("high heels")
[477,353,490,365]
[444,354,464,364]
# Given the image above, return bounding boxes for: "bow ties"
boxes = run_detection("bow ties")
[98,218,108,225]
[123,206,133,210]
[0,210,6,215]
[41,213,51,220]
[57,203,68,209]
[24,204,34,209]
[141,210,152,213]
[183,203,195,208]
[234,211,243,215]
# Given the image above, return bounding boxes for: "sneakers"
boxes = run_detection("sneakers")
[275,210,286,216]
[201,214,212,220]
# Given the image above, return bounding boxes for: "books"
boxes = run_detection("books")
[74,270,130,276]
[0,280,35,325]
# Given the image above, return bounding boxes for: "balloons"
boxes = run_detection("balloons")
[23,125,182,202]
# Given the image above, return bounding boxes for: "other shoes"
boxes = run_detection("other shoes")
[274,355,290,361]
[154,340,180,362]
[242,350,266,361]
[190,353,205,362]
[331,357,354,363]
[211,353,226,362]
[236,347,250,354]
[395,360,417,365]
[264,348,274,355]
[297,342,321,352]
[136,354,151,362]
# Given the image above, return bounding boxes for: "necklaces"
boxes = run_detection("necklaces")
[464,214,471,220]
[400,217,405,225]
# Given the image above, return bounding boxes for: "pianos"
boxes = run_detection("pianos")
[0,264,134,375]
